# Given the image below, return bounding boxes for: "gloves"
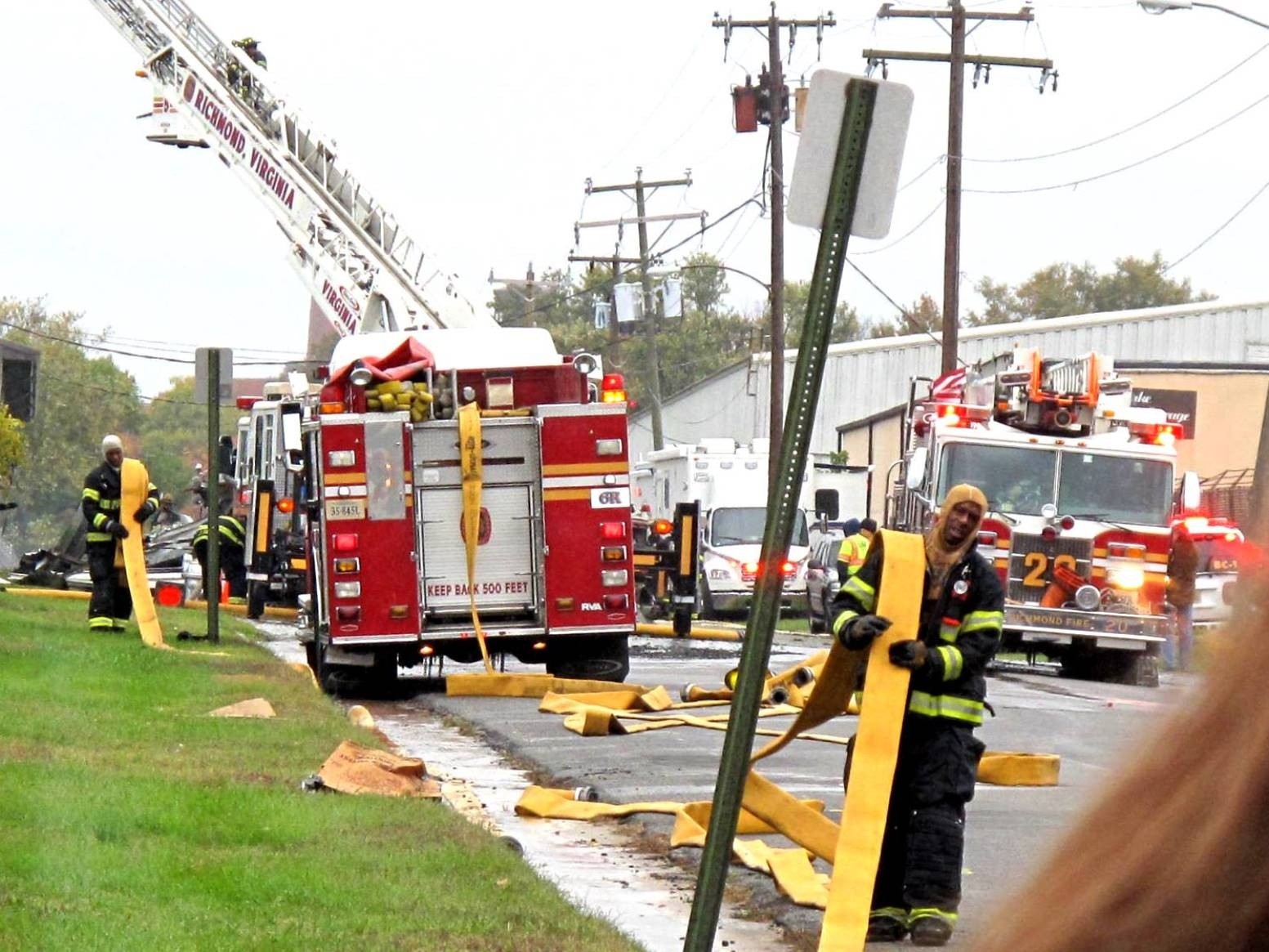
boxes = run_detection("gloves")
[133,502,156,524]
[844,614,891,651]
[108,523,129,540]
[888,640,933,669]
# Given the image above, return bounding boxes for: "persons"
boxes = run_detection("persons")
[151,492,182,567]
[836,517,877,588]
[190,515,247,605]
[832,482,1006,946]
[81,435,159,633]
[1162,523,1198,670]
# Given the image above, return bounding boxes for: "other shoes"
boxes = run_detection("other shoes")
[865,915,908,943]
[910,917,954,947]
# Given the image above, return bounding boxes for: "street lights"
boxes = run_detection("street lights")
[1137,0,1269,30]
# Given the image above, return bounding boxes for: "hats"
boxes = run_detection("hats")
[1172,522,1189,537]
[928,483,988,558]
[101,435,124,472]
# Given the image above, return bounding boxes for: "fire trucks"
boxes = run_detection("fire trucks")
[92,1,1245,698]
